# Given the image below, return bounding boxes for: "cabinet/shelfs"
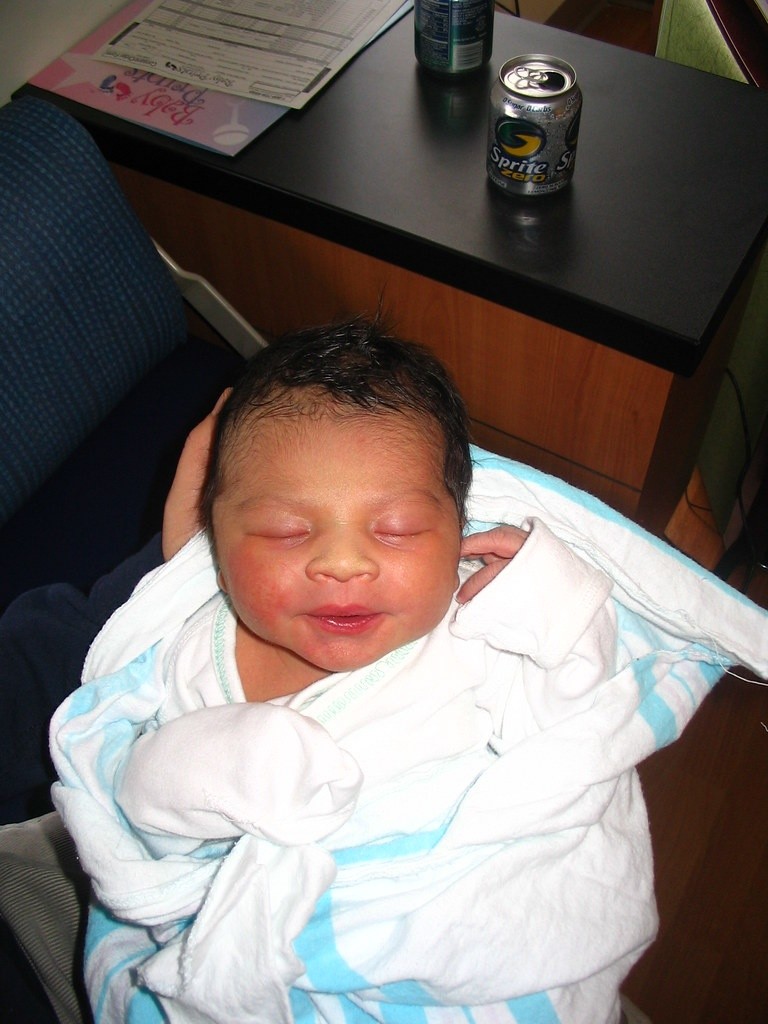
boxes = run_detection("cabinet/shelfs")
[10,7,768,537]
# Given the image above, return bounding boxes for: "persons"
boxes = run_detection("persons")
[49,296,767,1024]
[0,388,235,1023]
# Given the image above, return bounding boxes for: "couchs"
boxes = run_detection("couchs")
[0,94,270,613]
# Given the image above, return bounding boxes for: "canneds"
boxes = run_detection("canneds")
[413,0,495,78]
[487,54,583,196]
